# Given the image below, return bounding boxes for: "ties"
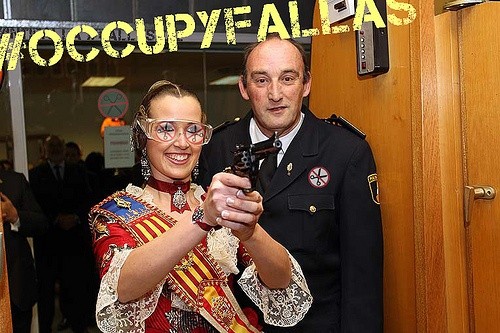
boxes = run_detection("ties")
[255,149,278,197]
[54,165,63,184]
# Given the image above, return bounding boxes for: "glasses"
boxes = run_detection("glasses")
[135,115,214,147]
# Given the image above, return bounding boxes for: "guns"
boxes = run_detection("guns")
[221,132,282,193]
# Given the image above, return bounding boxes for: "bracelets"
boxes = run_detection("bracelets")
[192,205,222,231]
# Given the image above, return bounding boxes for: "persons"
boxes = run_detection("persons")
[0,135,146,333]
[190,31,384,333]
[88,81,313,333]
